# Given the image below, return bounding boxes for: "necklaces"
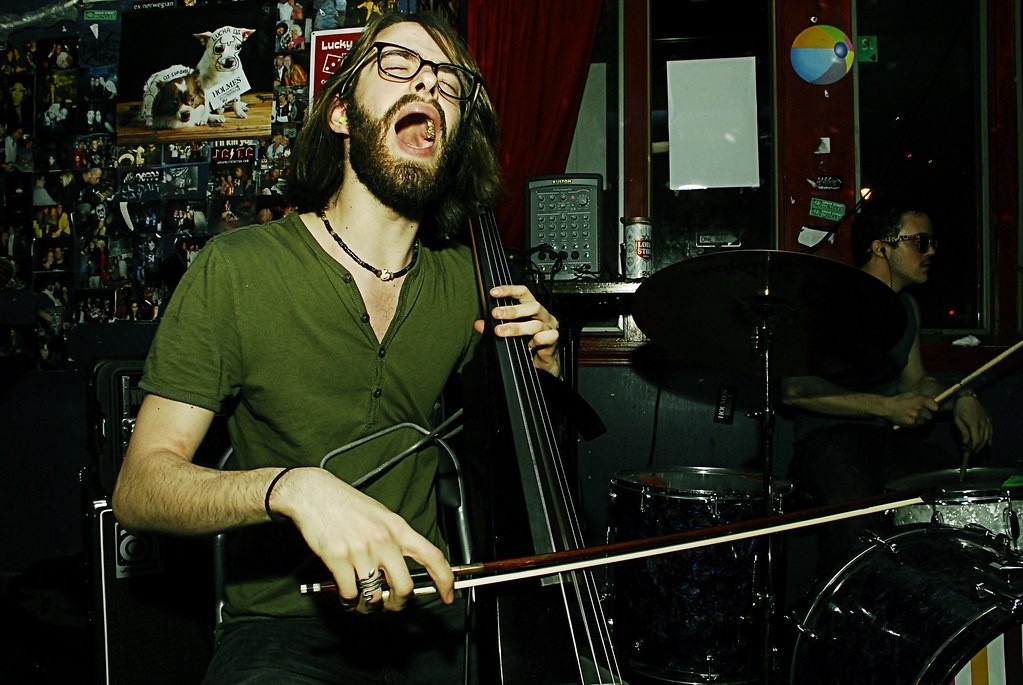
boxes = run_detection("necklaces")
[321,210,418,281]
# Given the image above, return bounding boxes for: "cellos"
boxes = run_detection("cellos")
[462,206,627,685]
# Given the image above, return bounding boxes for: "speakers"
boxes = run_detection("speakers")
[87,496,228,685]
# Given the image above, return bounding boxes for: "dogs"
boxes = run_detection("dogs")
[133,26,257,127]
[151,67,208,131]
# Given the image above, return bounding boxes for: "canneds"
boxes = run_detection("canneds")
[623,217,652,279]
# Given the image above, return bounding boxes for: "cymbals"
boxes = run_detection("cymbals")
[630,247,910,379]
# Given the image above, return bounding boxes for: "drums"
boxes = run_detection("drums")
[882,464,1023,559]
[599,464,804,685]
[772,520,1023,685]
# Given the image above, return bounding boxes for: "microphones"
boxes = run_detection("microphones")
[880,247,888,260]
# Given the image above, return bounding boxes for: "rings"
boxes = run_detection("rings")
[359,569,382,606]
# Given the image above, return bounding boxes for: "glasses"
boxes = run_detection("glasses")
[339,42,484,130]
[865,232,939,255]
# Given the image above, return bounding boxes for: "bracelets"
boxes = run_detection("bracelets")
[957,389,977,398]
[264,466,293,521]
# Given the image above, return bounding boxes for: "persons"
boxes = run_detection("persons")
[0,0,315,354]
[112,12,562,685]
[780,206,993,496]
